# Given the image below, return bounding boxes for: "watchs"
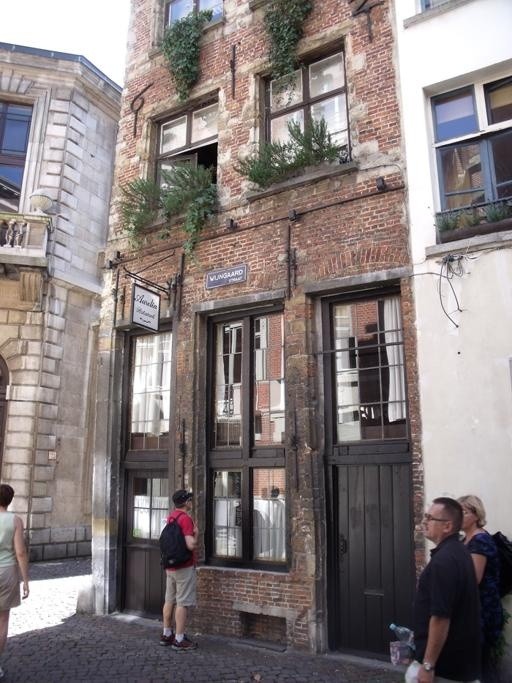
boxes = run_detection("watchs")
[422,661,435,671]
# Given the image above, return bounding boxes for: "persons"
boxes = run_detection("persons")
[0,484,30,679]
[412,496,485,683]
[454,495,504,682]
[159,489,199,651]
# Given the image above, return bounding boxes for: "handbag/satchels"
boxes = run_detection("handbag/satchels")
[491,528,512,598]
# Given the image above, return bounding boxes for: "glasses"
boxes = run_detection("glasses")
[423,511,452,522]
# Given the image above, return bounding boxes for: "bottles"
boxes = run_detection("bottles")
[389,622,416,652]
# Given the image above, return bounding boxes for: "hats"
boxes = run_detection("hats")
[172,487,194,508]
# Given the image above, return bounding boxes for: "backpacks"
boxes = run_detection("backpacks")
[157,511,193,571]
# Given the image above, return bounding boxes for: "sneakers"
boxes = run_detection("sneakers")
[171,634,200,650]
[159,631,176,646]
